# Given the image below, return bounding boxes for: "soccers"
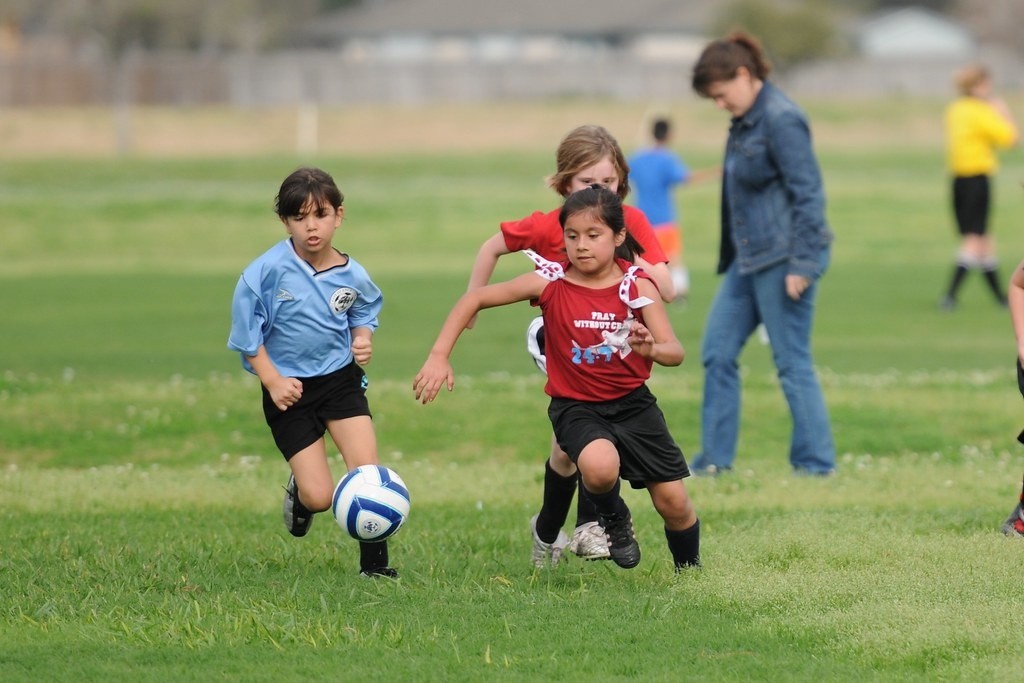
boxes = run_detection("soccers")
[330,463,412,543]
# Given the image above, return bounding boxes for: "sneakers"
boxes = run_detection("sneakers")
[596,497,641,569]
[530,513,570,568]
[570,520,611,559]
[1001,502,1024,537]
[359,565,398,579]
[672,556,702,574]
[281,472,313,538]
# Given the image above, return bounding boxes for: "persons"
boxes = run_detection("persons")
[412,183,702,573]
[939,66,1018,310]
[465,125,676,570]
[625,120,723,297]
[1001,259,1024,537]
[227,167,398,580]
[685,33,836,475]
